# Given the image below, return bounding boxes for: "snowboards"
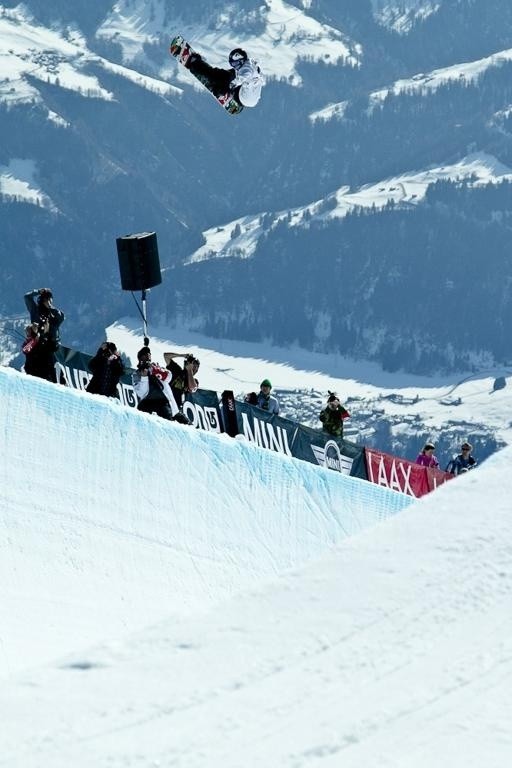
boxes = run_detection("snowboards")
[169,37,243,114]
[324,440,342,472]
[22,311,65,356]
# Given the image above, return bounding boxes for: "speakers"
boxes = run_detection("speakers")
[115,230,162,291]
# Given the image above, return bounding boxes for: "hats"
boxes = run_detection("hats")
[261,379,272,389]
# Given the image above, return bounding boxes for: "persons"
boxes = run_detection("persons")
[450,443,477,475]
[86,342,124,398]
[132,347,173,420]
[417,445,439,468]
[226,49,263,111]
[164,352,200,425]
[24,289,64,381]
[320,396,349,438]
[255,379,279,413]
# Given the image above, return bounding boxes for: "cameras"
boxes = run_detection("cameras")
[104,349,114,358]
[188,356,195,363]
[137,360,151,372]
[251,392,260,405]
[37,293,52,303]
